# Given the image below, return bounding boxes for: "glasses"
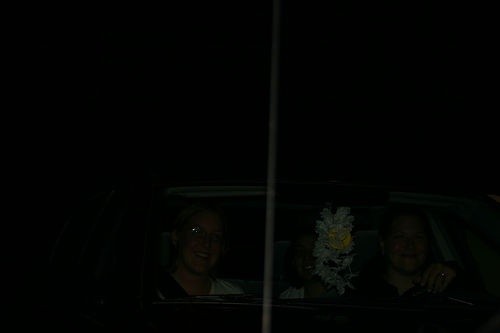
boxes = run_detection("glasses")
[185,225,224,244]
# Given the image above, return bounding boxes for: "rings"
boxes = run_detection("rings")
[440,272,447,279]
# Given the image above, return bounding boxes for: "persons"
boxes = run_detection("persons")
[280,228,459,299]
[128,201,249,300]
[346,202,453,306]
